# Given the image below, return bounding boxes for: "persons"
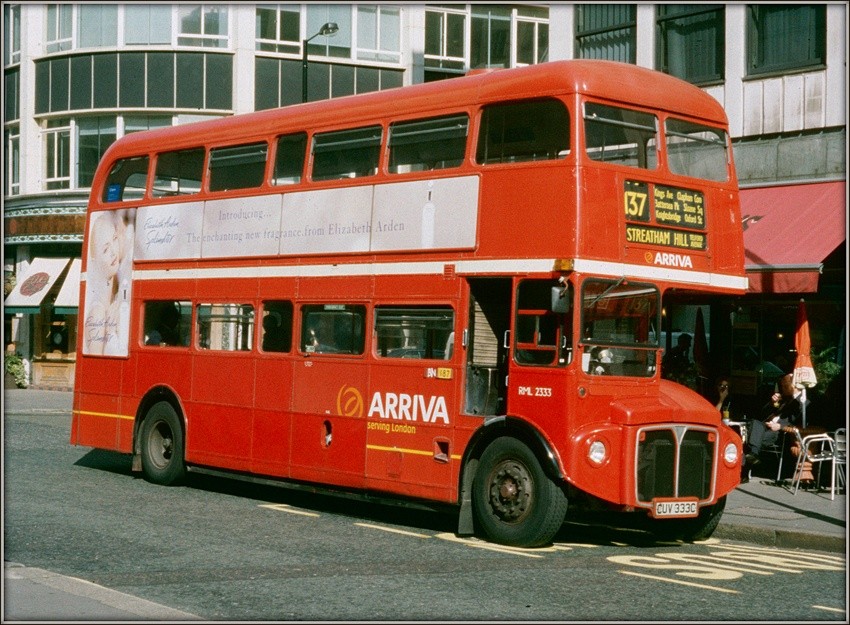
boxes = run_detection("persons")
[263,315,292,353]
[79,209,129,357]
[661,333,846,482]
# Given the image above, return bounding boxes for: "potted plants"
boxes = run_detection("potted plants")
[4,350,26,389]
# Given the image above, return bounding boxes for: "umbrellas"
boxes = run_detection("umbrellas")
[692,306,710,399]
[791,298,819,489]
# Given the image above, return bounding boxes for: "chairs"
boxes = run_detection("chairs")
[791,426,846,501]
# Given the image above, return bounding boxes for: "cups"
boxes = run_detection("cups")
[774,393,781,407]
[723,407,729,419]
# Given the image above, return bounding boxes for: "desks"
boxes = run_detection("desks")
[777,425,823,481]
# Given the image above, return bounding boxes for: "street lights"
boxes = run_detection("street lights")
[301,22,341,103]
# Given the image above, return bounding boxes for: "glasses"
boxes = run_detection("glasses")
[720,384,729,389]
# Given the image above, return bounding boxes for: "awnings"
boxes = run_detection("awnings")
[53,256,82,315]
[733,176,848,293]
[5,255,72,314]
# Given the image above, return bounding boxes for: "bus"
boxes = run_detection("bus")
[69,58,750,551]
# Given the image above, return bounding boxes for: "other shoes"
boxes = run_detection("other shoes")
[745,453,761,465]
[741,475,748,483]
